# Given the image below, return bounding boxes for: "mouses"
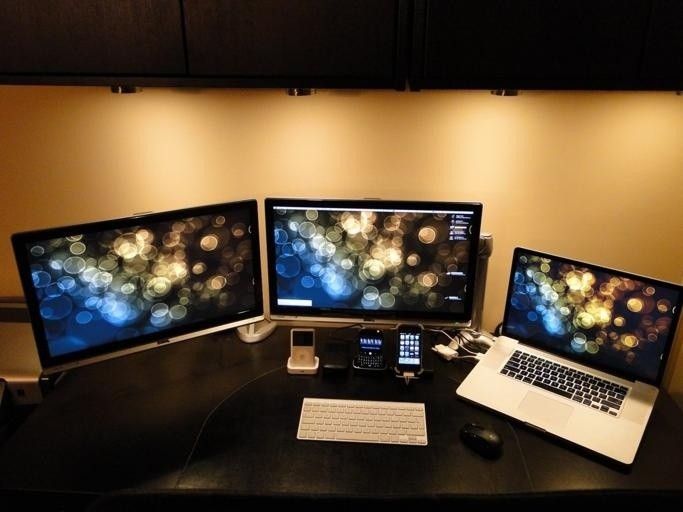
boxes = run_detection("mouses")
[458,422,503,462]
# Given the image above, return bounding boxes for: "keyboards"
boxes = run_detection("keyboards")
[295,398,429,446]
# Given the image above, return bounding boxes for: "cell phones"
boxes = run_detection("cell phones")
[395,323,423,377]
[357,328,384,369]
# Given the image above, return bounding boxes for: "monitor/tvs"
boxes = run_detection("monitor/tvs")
[8,198,262,372]
[266,197,483,328]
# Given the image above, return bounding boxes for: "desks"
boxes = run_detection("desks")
[0,295,682,511]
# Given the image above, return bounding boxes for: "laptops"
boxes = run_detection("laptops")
[454,248,683,467]
